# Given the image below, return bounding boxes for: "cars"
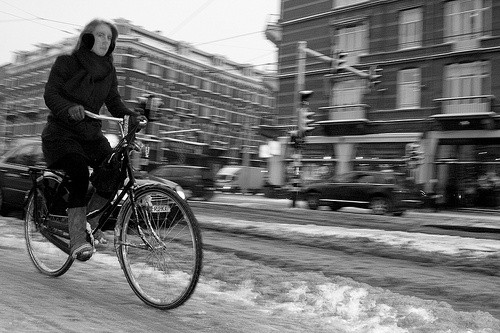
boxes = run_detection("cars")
[305,171,425,217]
[0,140,187,232]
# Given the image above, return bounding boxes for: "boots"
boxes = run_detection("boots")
[66,207,93,258]
[86,192,109,243]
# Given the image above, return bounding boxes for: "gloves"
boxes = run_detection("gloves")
[68,105,84,122]
[136,115,148,122]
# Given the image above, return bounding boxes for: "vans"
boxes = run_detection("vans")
[152,165,215,201]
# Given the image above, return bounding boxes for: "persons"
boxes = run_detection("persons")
[41,19,147,262]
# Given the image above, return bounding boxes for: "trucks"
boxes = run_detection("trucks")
[215,165,266,195]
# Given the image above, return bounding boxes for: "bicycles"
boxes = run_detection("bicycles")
[24,110,204,311]
